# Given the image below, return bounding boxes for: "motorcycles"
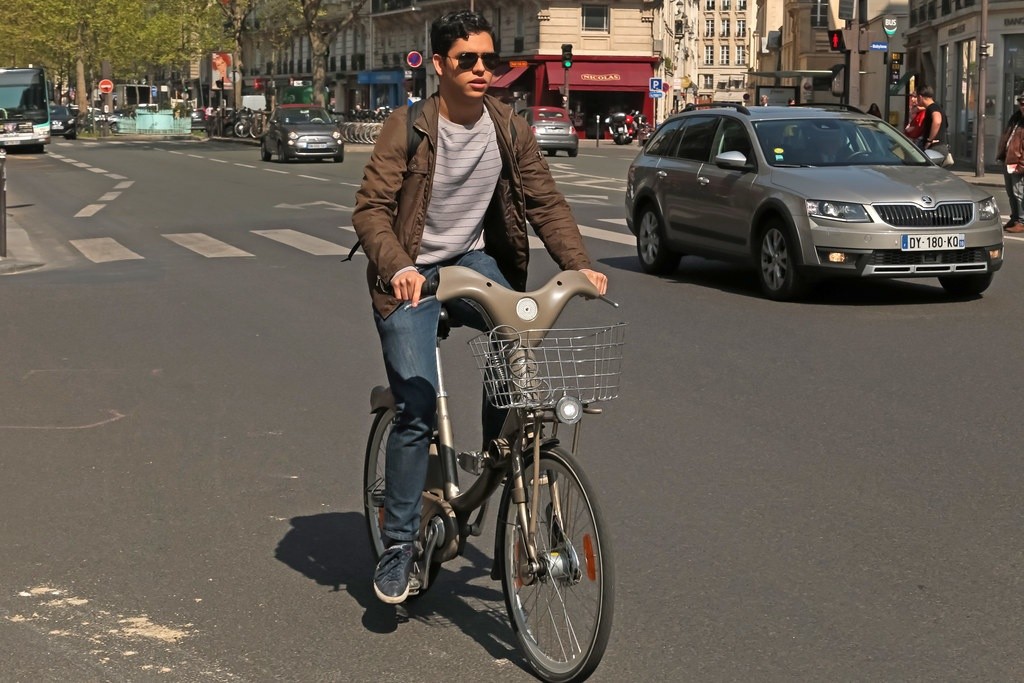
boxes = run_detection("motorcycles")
[605,111,637,144]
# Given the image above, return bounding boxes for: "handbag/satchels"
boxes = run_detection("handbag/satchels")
[939,153,954,168]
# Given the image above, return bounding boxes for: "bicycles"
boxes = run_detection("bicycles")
[363,265,628,683]
[216,108,265,139]
[335,109,393,144]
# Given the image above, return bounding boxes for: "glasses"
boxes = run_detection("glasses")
[1020,102,1024,106]
[442,52,501,69]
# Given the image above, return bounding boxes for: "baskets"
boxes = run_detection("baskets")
[467,321,628,407]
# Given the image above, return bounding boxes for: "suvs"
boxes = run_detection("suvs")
[48,105,77,140]
[67,104,122,133]
[259,103,344,164]
[186,108,213,137]
[624,100,1007,302]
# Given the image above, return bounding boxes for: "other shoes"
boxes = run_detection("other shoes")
[1003,220,1019,230]
[1006,223,1024,232]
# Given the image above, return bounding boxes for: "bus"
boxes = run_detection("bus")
[0,67,52,154]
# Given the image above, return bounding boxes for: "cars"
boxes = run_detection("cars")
[518,105,580,157]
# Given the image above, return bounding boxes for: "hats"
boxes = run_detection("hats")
[1016,92,1024,102]
[909,91,917,96]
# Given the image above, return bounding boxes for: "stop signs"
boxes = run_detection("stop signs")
[99,78,114,94]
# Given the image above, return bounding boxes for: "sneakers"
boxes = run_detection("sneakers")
[372,540,418,603]
[500,473,549,485]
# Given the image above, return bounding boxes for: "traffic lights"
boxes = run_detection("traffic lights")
[826,26,853,55]
[562,42,574,73]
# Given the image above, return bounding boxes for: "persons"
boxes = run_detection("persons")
[812,134,841,162]
[867,103,881,118]
[760,95,770,106]
[351,11,608,602]
[788,97,795,104]
[904,91,926,151]
[997,93,1024,232]
[917,86,948,168]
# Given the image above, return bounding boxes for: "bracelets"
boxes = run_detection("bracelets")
[927,137,933,142]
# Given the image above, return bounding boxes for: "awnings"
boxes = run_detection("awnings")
[489,63,529,88]
[546,61,653,90]
